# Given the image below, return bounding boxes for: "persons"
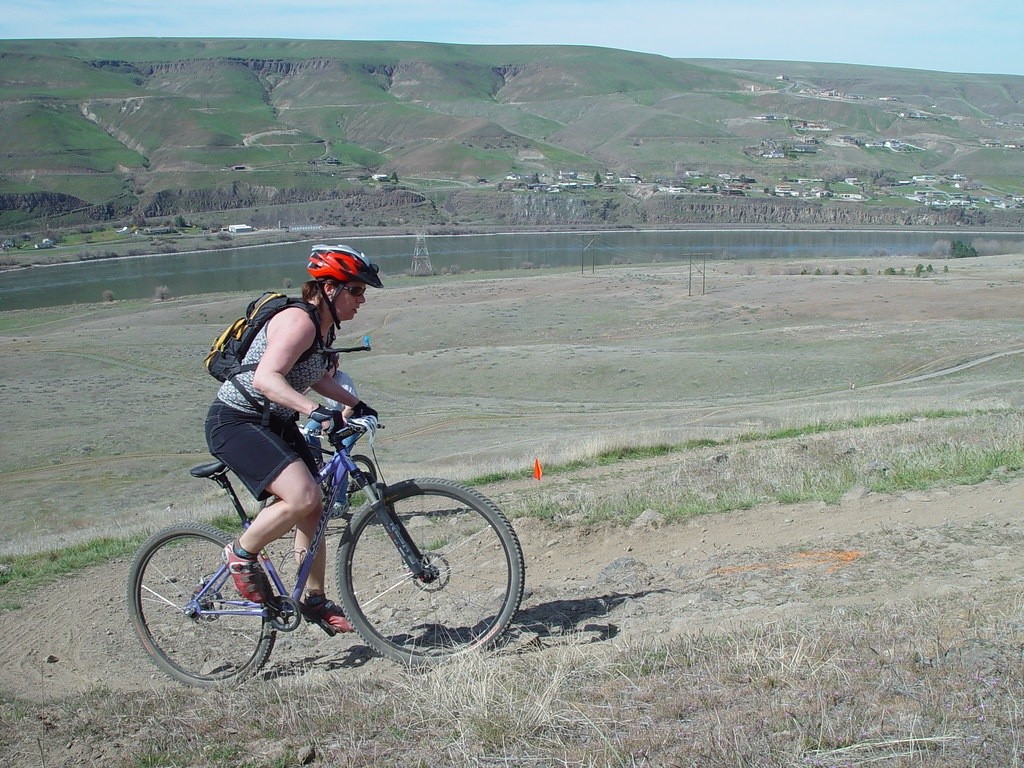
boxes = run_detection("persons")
[204,243,384,633]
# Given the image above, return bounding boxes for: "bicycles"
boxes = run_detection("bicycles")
[127,410,527,689]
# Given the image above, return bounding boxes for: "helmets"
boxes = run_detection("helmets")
[306,243,384,288]
[330,347,339,362]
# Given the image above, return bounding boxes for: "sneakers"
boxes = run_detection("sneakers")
[303,593,355,634]
[330,500,349,519]
[221,540,274,603]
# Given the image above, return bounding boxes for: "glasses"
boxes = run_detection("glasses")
[343,286,366,297]
[329,363,336,369]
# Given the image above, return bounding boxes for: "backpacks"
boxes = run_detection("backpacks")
[203,291,321,383]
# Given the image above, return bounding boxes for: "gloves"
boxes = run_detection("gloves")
[351,400,379,421]
[307,403,347,436]
